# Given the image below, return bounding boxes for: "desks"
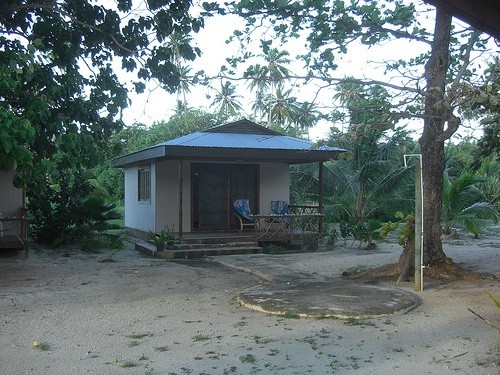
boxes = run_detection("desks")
[254,213,324,240]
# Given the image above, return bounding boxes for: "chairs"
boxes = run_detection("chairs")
[231,198,260,232]
[264,200,291,232]
